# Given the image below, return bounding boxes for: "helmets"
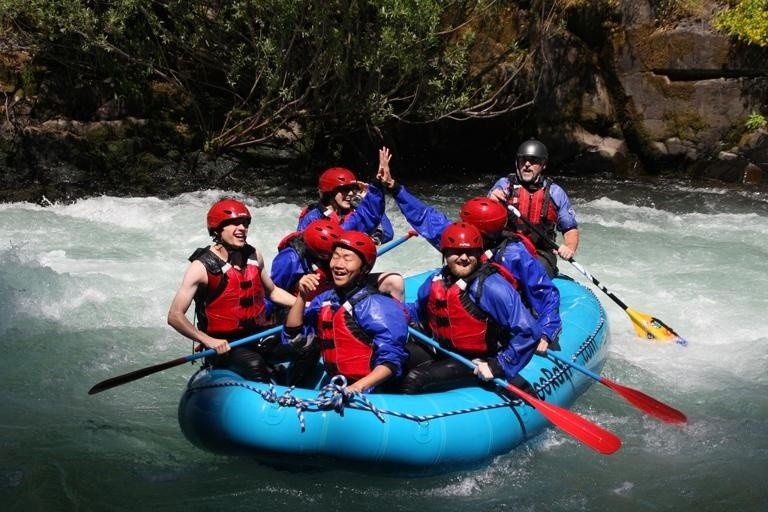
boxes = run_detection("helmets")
[318,167,359,206]
[460,196,508,240]
[206,199,252,244]
[332,230,377,283]
[303,219,344,262]
[515,139,549,183]
[439,221,483,252]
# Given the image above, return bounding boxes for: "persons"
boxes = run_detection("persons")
[296,167,406,302]
[271,146,392,301]
[382,152,562,353]
[281,232,410,398]
[379,222,542,396]
[167,199,318,386]
[487,140,579,279]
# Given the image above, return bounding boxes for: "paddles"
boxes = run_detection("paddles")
[408,325,621,455]
[88,326,285,395]
[545,348,687,424]
[499,199,688,347]
